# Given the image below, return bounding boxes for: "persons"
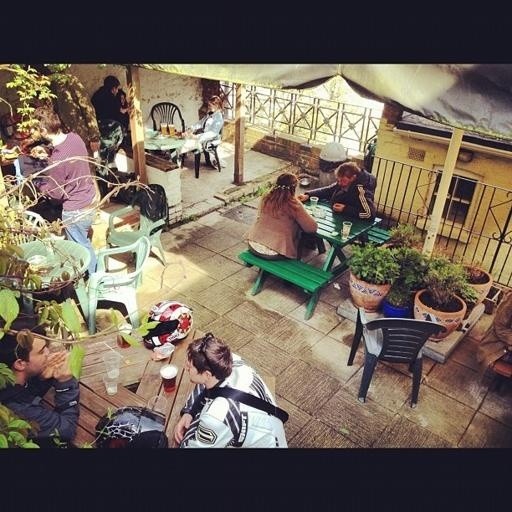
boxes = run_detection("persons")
[247,172,318,259]
[170,95,223,168]
[173,333,287,448]
[298,161,377,219]
[1,316,80,448]
[89,75,131,162]
[30,106,101,281]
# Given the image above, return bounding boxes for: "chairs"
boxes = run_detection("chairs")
[348,307,447,408]
[106,184,168,283]
[15,211,46,245]
[151,103,185,132]
[208,129,222,172]
[88,236,151,335]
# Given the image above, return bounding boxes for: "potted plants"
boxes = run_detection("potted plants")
[346,223,494,342]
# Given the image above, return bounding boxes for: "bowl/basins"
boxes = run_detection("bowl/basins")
[298,177,311,188]
[28,255,47,271]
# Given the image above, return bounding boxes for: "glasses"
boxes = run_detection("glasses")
[197,333,215,376]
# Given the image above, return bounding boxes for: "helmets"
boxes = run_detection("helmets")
[97,405,167,450]
[143,300,194,350]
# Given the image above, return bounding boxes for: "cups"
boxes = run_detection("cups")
[341,221,352,239]
[160,364,179,394]
[103,352,121,396]
[160,122,176,138]
[309,196,319,211]
[148,396,167,416]
[116,322,133,348]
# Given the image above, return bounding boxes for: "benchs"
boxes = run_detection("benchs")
[108,147,181,207]
[238,251,334,320]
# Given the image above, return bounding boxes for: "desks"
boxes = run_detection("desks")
[144,129,202,178]
[40,329,206,448]
[302,197,383,276]
